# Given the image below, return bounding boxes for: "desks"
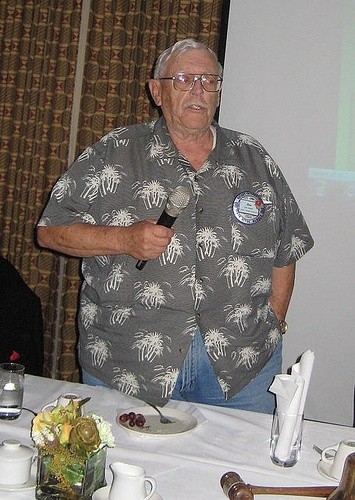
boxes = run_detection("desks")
[0,367,355,500]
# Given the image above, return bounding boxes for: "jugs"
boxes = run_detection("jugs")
[110,462,157,500]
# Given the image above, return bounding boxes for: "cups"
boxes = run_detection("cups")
[0,363,26,421]
[42,393,84,417]
[322,439,355,480]
[270,407,304,467]
[0,439,34,486]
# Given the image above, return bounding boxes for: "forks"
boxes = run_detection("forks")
[148,401,175,424]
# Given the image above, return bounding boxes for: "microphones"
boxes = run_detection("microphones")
[136,185,191,271]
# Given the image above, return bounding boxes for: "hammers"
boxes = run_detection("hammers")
[220,470,338,500]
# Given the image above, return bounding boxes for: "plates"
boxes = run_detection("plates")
[0,462,38,491]
[115,406,198,436]
[317,457,340,482]
[92,486,163,500]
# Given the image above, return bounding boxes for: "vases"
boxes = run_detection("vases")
[33,445,108,500]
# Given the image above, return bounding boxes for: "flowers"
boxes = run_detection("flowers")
[30,396,115,495]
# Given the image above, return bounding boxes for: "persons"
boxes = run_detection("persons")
[37,39,314,415]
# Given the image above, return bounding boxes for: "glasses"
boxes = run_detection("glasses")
[159,73,223,93]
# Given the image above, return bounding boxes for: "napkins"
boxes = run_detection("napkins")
[269,350,315,461]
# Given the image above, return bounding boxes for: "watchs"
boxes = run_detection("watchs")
[278,320,288,334]
